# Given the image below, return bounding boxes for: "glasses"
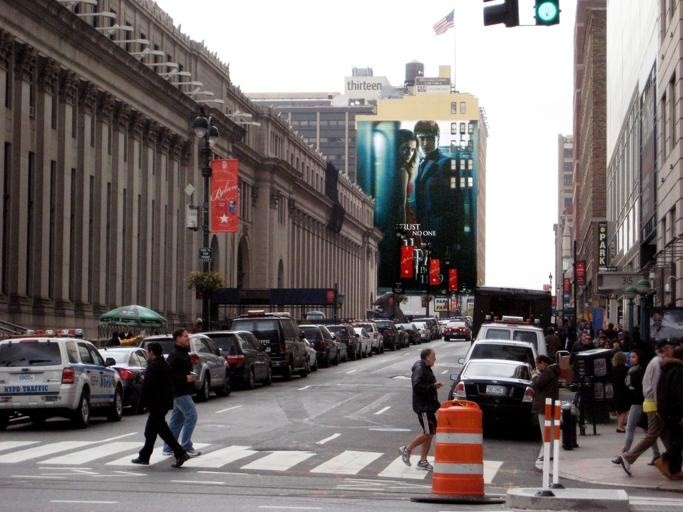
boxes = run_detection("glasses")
[584,336,593,339]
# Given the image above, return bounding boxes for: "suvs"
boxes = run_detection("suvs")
[443,316,471,342]
[0,327,125,429]
[231,309,319,382]
[372,319,403,351]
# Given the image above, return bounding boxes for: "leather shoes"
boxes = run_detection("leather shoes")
[616,427,626,434]
[131,458,152,466]
[171,452,190,469]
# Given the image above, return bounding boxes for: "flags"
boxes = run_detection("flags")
[433,10,455,34]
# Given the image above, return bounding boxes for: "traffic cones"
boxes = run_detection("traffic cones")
[409,399,504,505]
[550,401,568,492]
[539,396,553,501]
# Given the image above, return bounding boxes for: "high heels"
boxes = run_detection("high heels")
[615,456,635,477]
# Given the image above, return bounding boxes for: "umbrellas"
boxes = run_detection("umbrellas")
[99,305,168,325]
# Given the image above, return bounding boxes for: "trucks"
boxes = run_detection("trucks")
[471,285,554,348]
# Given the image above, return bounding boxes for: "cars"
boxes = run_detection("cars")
[456,339,540,378]
[352,327,375,358]
[97,346,151,415]
[449,357,541,441]
[137,332,235,401]
[202,331,275,390]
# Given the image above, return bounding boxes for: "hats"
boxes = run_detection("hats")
[655,337,679,348]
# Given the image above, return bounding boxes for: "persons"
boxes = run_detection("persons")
[378,128,422,285]
[655,345,683,480]
[611,352,633,433]
[611,350,662,465]
[619,341,674,476]
[107,332,120,347]
[162,328,201,459]
[131,342,190,469]
[414,121,452,281]
[565,316,634,401]
[544,327,562,359]
[399,348,444,472]
[650,313,669,340]
[530,355,560,461]
[119,326,133,340]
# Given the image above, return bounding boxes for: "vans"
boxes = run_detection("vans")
[354,323,385,355]
[412,317,443,340]
[471,316,549,362]
[297,323,349,368]
[395,323,423,348]
[410,322,432,343]
[325,323,363,362]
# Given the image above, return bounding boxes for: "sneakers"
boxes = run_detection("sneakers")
[416,459,434,474]
[162,450,174,458]
[185,449,203,458]
[673,474,679,480]
[399,445,413,468]
[653,455,672,480]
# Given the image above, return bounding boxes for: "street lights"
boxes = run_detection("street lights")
[193,111,220,334]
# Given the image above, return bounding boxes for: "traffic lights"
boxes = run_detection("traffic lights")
[532,0,561,27]
[481,0,520,27]
